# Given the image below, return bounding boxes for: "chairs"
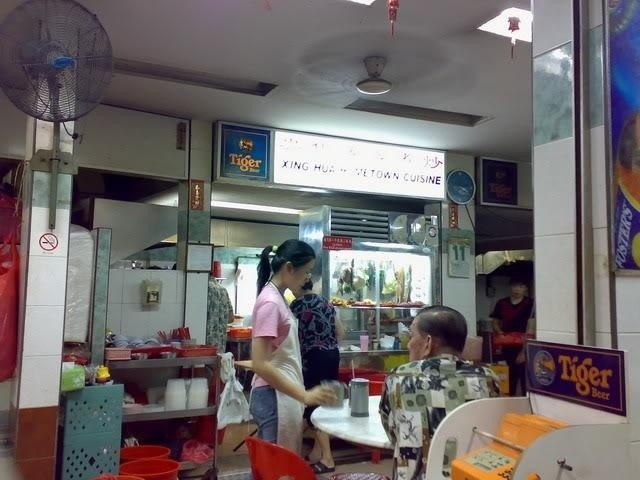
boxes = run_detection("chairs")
[244,434,318,480]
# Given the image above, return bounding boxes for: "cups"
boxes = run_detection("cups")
[360,336,368,350]
[321,380,344,410]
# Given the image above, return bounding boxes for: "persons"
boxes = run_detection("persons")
[379,303,502,480]
[206,272,237,372]
[248,236,338,478]
[290,272,341,476]
[488,270,537,399]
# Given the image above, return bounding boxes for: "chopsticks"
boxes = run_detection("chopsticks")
[157,327,190,341]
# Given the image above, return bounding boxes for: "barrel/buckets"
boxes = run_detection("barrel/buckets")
[91,445,181,480]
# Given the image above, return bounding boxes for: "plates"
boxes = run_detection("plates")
[123,403,163,414]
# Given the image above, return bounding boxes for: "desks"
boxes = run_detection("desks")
[310,395,394,452]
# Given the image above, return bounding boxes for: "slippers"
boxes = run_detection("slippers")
[311,462,335,473]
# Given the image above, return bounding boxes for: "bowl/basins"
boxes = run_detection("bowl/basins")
[165,377,209,409]
[131,353,151,360]
[160,352,178,358]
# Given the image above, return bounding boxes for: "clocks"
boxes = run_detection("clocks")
[445,169,476,205]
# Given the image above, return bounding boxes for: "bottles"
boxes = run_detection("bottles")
[351,378,370,417]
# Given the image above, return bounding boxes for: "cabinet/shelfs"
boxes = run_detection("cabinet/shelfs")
[107,355,221,471]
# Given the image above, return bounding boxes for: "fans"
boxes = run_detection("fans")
[0,0,114,230]
[293,54,456,109]
